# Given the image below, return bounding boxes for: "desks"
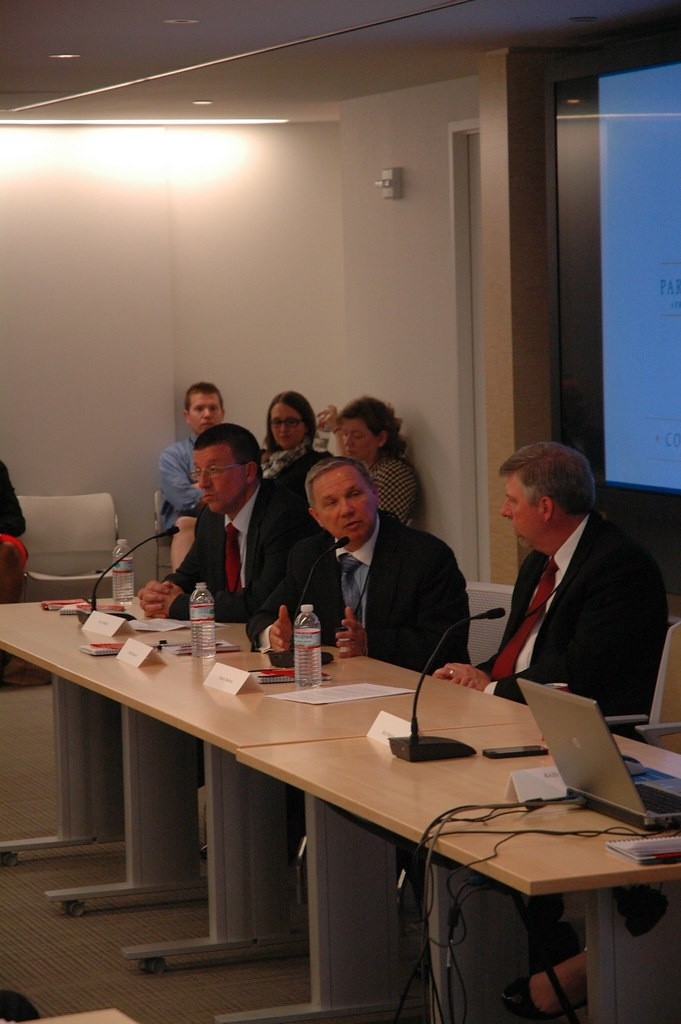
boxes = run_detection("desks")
[0,597,680,1024]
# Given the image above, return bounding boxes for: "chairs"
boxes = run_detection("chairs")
[170,517,197,573]
[17,493,119,602]
[466,581,515,667]
[604,622,680,754]
[154,491,178,580]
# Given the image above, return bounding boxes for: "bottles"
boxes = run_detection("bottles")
[293,604,323,690]
[188,582,216,659]
[112,539,134,606]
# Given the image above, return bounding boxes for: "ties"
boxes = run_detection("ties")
[492,557,559,681]
[339,554,362,624]
[224,522,242,592]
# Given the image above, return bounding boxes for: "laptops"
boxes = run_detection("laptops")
[516,678,681,831]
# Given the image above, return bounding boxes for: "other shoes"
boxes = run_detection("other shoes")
[500,966,587,1019]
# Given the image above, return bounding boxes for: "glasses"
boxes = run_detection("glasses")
[272,418,305,427]
[189,463,242,482]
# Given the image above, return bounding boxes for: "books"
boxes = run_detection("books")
[59,604,124,615]
[162,639,241,654]
[80,643,126,655]
[250,669,332,683]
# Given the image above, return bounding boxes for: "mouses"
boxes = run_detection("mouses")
[621,753,647,776]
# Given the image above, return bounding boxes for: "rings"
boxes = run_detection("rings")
[352,641,355,648]
[449,669,455,679]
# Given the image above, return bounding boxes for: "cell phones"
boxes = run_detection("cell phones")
[482,745,549,759]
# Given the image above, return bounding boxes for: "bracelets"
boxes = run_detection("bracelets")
[332,428,344,434]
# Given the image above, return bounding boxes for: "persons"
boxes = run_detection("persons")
[260,391,347,499]
[0,459,28,686]
[155,382,225,549]
[138,424,324,623]
[338,396,425,527]
[432,443,669,743]
[245,455,470,676]
[501,948,587,1020]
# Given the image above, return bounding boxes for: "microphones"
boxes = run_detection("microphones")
[268,536,351,668]
[387,607,506,762]
[75,526,180,624]
